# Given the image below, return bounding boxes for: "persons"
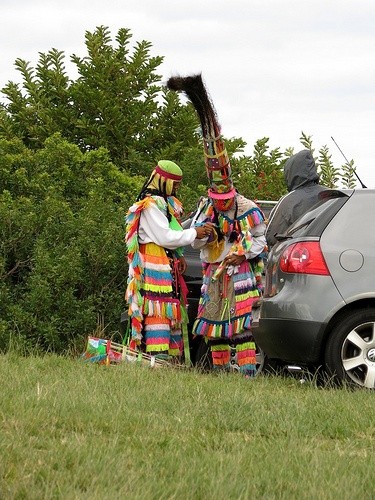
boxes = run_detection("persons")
[264,149,329,383]
[126,159,206,362]
[190,184,266,379]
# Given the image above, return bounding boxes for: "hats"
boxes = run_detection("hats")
[145,159,183,197]
[165,76,238,199]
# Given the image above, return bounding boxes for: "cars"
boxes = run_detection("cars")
[249,187,375,394]
[167,200,279,381]
